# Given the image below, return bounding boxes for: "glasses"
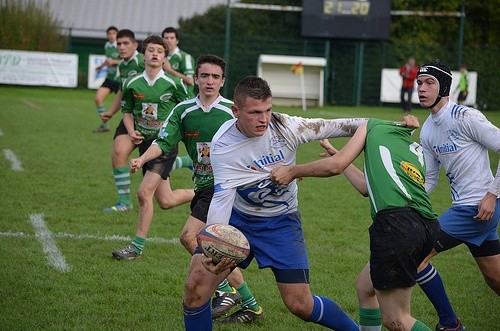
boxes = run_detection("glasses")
[117,41,129,45]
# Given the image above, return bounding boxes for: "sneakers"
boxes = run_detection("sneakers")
[222,306,263,325]
[211,286,242,318]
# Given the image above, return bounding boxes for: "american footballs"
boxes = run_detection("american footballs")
[197,224,251,263]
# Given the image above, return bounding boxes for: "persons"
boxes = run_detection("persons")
[179,75,421,331]
[272,117,484,331]
[453,61,469,105]
[91,25,264,324]
[399,57,418,112]
[413,58,500,331]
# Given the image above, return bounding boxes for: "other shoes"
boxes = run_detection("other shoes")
[435,319,465,331]
[103,203,133,213]
[112,246,142,260]
[97,125,110,132]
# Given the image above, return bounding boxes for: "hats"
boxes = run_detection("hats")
[460,64,467,67]
